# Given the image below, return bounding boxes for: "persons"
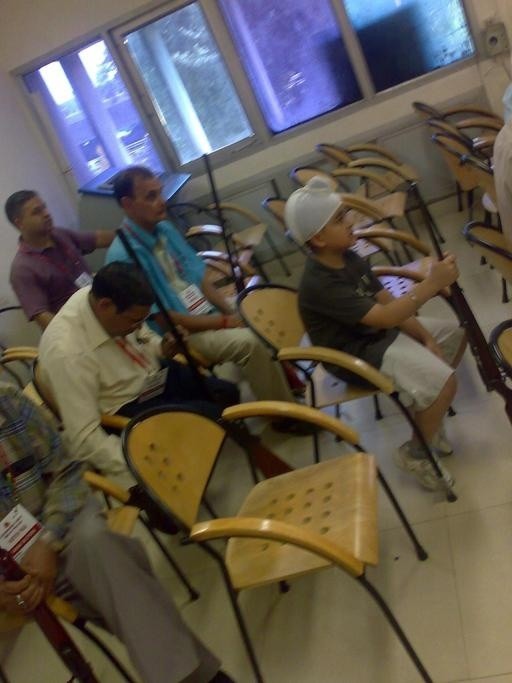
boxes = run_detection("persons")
[283,176,468,490]
[37,261,242,533]
[103,167,320,435]
[0,381,233,683]
[5,189,115,329]
[493,83,512,241]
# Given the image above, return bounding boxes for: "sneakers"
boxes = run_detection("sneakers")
[428,432,453,457]
[268,412,325,436]
[392,439,456,492]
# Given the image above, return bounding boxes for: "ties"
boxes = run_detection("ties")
[115,335,155,373]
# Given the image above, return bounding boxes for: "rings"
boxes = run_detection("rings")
[17,595,24,607]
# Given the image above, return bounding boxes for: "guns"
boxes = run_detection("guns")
[203,154,307,393]
[0,546,100,683]
[116,226,293,478]
[408,179,512,423]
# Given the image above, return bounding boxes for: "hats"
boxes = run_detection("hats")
[281,175,344,248]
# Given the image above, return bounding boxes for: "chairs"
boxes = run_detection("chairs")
[0,99,512,683]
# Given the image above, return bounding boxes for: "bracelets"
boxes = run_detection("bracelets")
[408,288,424,308]
